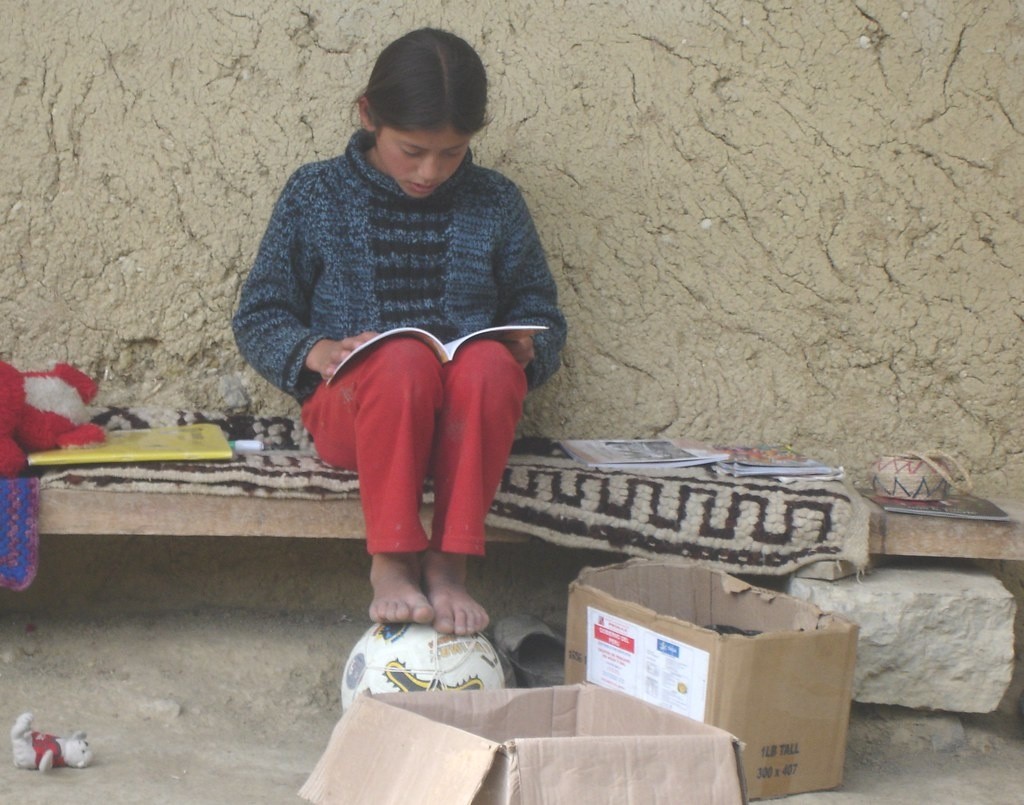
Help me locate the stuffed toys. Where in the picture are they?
[10,711,95,774]
[0,362,108,476]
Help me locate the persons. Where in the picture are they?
[233,26,568,641]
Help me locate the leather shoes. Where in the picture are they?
[492,612,565,686]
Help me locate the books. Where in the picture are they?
[557,434,845,485]
[24,421,233,467]
[323,316,550,393]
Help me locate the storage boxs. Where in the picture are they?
[561,558,860,798]
[293,682,753,804]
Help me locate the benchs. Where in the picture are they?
[1,441,1021,588]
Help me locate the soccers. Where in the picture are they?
[341,622,505,715]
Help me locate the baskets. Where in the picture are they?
[872,451,949,502]
